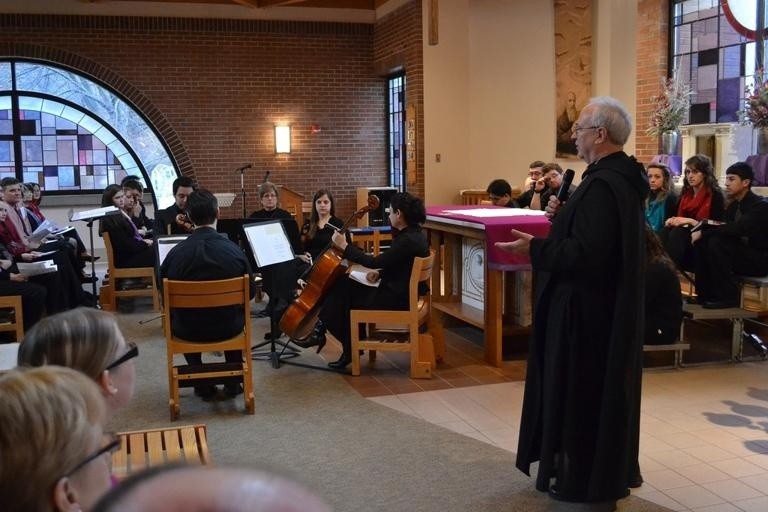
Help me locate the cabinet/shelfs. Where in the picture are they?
[356,187,398,229]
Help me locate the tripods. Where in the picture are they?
[251,273,301,353]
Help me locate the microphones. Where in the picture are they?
[236,165,253,170]
[556,169,575,202]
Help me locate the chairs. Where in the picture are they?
[0,295,27,344]
[348,247,447,380]
[163,273,256,424]
[98,230,160,313]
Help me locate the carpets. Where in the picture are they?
[0,262,683,511]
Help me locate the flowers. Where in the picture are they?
[642,50,698,140]
[734,52,768,136]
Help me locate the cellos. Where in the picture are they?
[278,194,379,340]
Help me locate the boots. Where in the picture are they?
[329,351,363,367]
[291,321,327,353]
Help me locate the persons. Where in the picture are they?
[528,162,578,209]
[664,153,725,275]
[515,160,547,208]
[493,96,652,504]
[486,178,520,208]
[644,154,678,255]
[686,161,768,309]
[557,92,584,155]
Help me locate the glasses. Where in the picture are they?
[107,342,138,370]
[576,125,597,133]
[67,432,122,475]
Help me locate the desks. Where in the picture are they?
[345,225,393,256]
[419,203,552,370]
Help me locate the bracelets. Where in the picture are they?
[534,188,541,193]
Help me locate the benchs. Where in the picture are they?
[110,424,214,483]
[641,187,767,371]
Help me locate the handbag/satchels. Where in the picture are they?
[695,230,737,306]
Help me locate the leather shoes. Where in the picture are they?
[83,255,100,261]
[81,274,98,283]
[223,385,242,395]
[195,384,216,394]
[551,486,591,502]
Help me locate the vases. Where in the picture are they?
[662,127,679,156]
[756,123,768,153]
